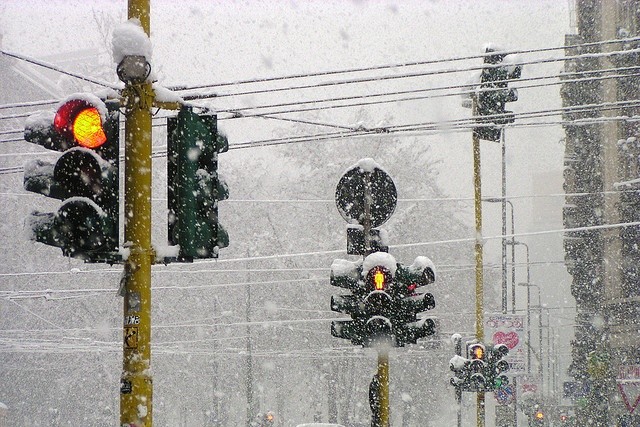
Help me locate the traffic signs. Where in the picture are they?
[484,313,524,331]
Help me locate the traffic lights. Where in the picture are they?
[395,265,435,347]
[24,112,65,250]
[330,264,363,345]
[53,95,119,263]
[474,48,520,141]
[363,265,396,346]
[467,345,488,390]
[489,344,509,390]
[450,355,467,389]
